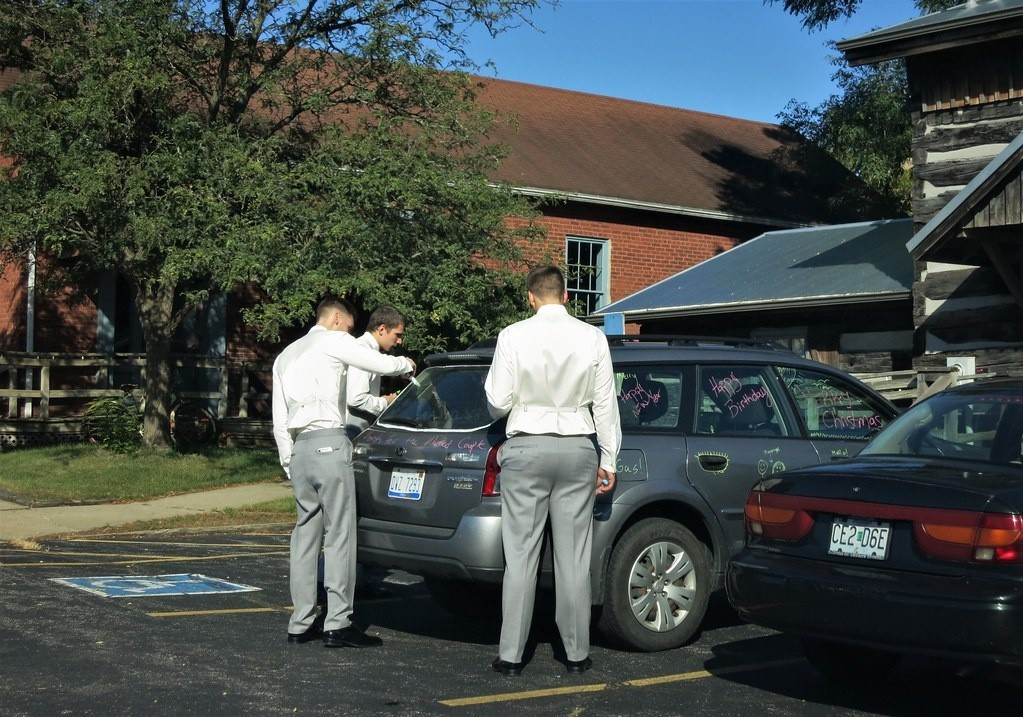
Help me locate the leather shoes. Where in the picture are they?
[323,626,383,649]
[288,620,324,643]
[568,656,593,674]
[492,657,522,677]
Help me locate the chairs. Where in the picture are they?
[731,383,781,434]
[620,376,668,425]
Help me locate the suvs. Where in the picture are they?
[353,334,1002,654]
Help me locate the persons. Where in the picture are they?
[272,296,417,649]
[345,305,407,442]
[484,265,622,677]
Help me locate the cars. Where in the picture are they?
[723,364,1023,675]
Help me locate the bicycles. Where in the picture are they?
[84,383,218,451]
